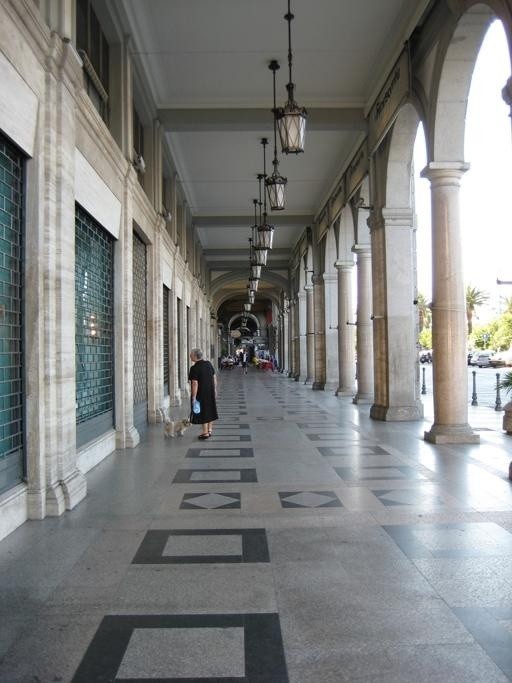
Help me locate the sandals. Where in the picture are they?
[199,431,212,439]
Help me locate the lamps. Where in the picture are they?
[243,0,308,312]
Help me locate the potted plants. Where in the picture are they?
[494,372,512,434]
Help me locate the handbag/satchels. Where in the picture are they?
[193,401,200,414]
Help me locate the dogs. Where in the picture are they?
[163,416,192,438]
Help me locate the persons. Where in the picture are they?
[240,349,248,375]
[187,347,218,441]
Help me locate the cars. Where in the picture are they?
[418,347,512,367]
